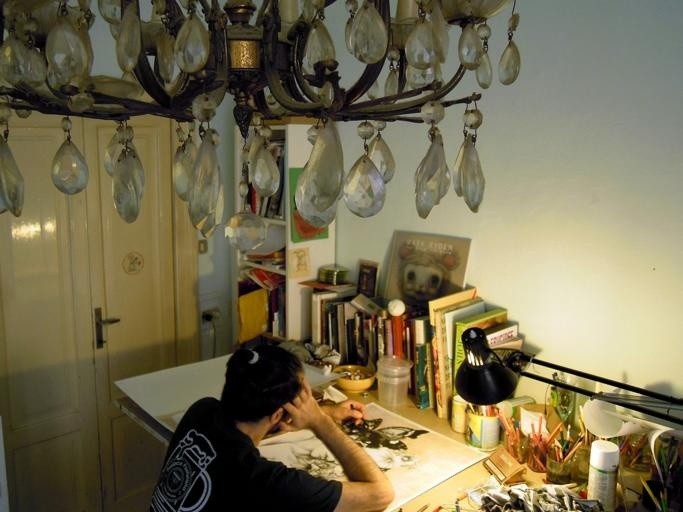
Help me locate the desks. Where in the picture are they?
[111,354,546,512]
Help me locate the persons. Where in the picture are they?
[148,337,395,511]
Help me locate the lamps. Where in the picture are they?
[454,327,683,432]
[0,1,521,255]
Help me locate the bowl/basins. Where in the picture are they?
[333,366,375,393]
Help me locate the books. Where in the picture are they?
[240,139,287,337]
[306,286,524,419]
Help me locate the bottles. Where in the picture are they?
[586,439,620,510]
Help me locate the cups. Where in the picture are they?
[450,396,575,485]
[375,375,409,408]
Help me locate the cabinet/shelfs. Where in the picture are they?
[232,117,335,343]
[0,75,203,511]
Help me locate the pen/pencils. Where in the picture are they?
[639,436,683,512]
[618,435,646,466]
[464,403,498,416]
[528,411,586,475]
[495,410,522,463]
[350,404,370,428]
